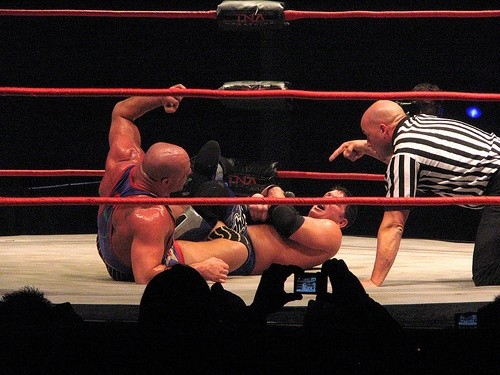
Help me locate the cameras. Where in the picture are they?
[294,273,327,294]
[455,312,477,326]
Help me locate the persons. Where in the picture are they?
[329,97,500,289]
[0,83,500,375]
